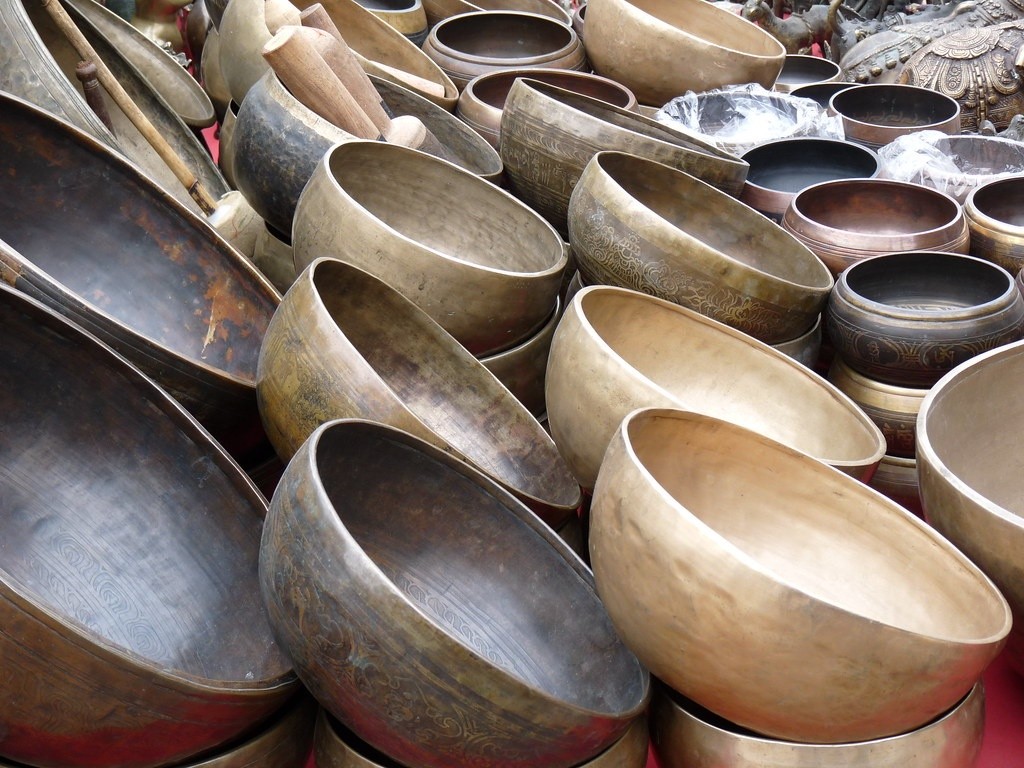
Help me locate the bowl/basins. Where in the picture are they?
[0,1,1023,767]
